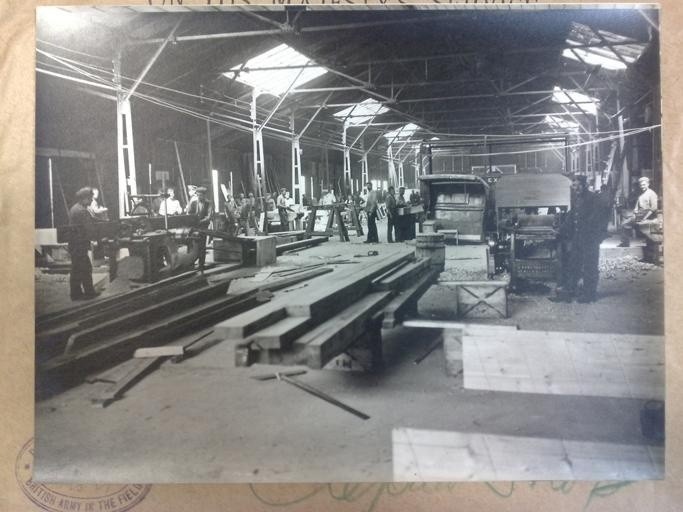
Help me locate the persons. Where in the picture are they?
[87,188,108,217]
[68,187,101,300]
[224,188,294,231]
[547,174,608,304]
[617,177,658,247]
[152,185,213,267]
[319,183,420,243]
